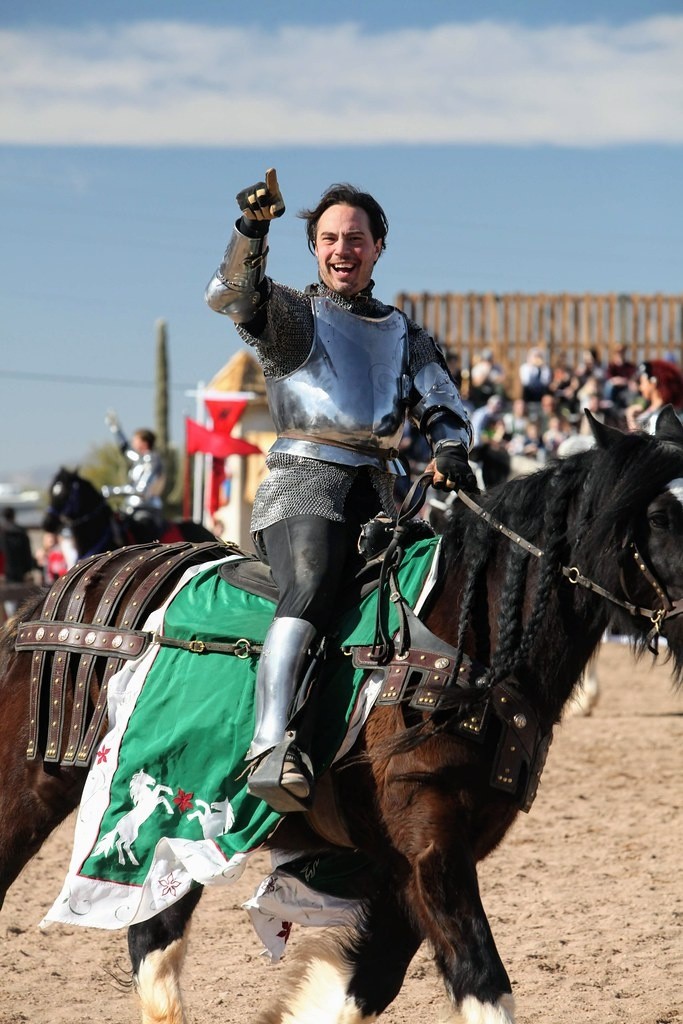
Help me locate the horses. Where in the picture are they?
[1,406,682,1024]
[40,465,219,564]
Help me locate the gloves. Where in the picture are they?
[235,166,286,224]
[425,448,479,494]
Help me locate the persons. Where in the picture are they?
[444,338,683,496]
[209,164,476,804]
[1,506,68,619]
[103,403,172,546]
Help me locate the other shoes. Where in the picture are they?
[247,745,310,799]
